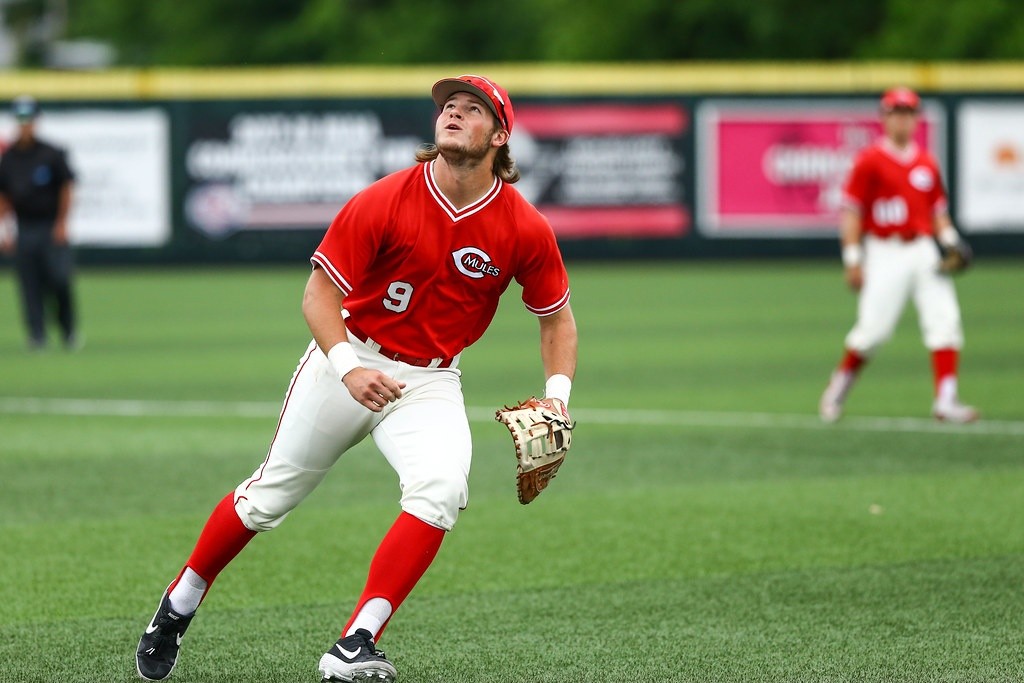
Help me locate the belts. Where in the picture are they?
[872,231,918,241]
[344,316,453,368]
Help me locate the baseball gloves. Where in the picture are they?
[936,242,974,276]
[496,397,574,507]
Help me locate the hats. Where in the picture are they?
[432,75,514,135]
[14,101,40,122]
[882,88,919,108]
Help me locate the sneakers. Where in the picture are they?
[319,628,397,683]
[136,578,197,682]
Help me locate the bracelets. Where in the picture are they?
[840,243,863,267]
[937,225,963,250]
[327,342,364,382]
[545,373,572,409]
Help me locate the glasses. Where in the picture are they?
[457,74,509,131]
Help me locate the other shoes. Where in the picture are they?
[820,368,852,422]
[932,402,978,422]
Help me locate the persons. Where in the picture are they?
[132,75,578,683]
[0,93,80,351]
[816,84,979,425]
